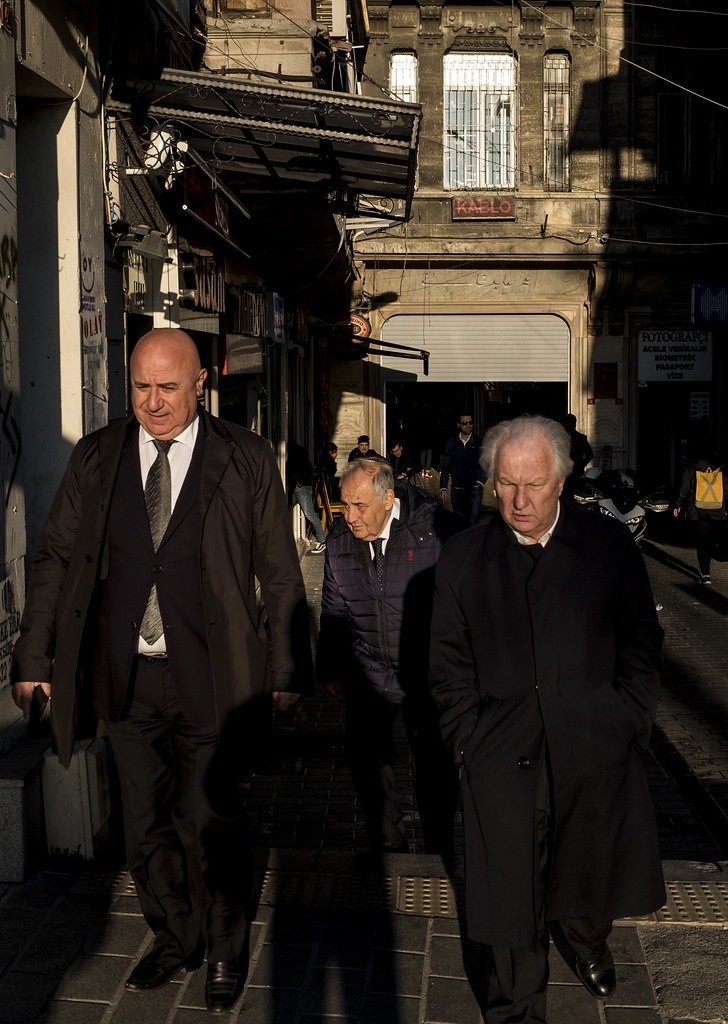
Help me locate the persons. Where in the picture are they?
[348,436,390,463]
[562,414,595,480]
[674,443,728,584]
[320,441,338,502]
[388,440,422,479]
[9,326,315,1016]
[317,457,473,878]
[427,415,667,1023]
[287,440,326,553]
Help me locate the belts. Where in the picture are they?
[138,654,167,662]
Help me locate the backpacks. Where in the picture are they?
[694,465,723,510]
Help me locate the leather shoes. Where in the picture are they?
[125,943,208,993]
[572,940,616,999]
[205,953,249,1016]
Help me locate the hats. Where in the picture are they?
[357,435,369,445]
[328,442,337,452]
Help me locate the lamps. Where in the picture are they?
[118,229,174,264]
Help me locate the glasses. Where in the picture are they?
[459,420,473,426]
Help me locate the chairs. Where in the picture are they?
[315,479,346,533]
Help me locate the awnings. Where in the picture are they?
[102,62,423,222]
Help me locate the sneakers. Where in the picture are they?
[697,573,712,584]
[310,540,327,554]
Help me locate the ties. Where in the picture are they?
[370,538,386,592]
[138,438,177,647]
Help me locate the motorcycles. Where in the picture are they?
[573,465,673,547]
